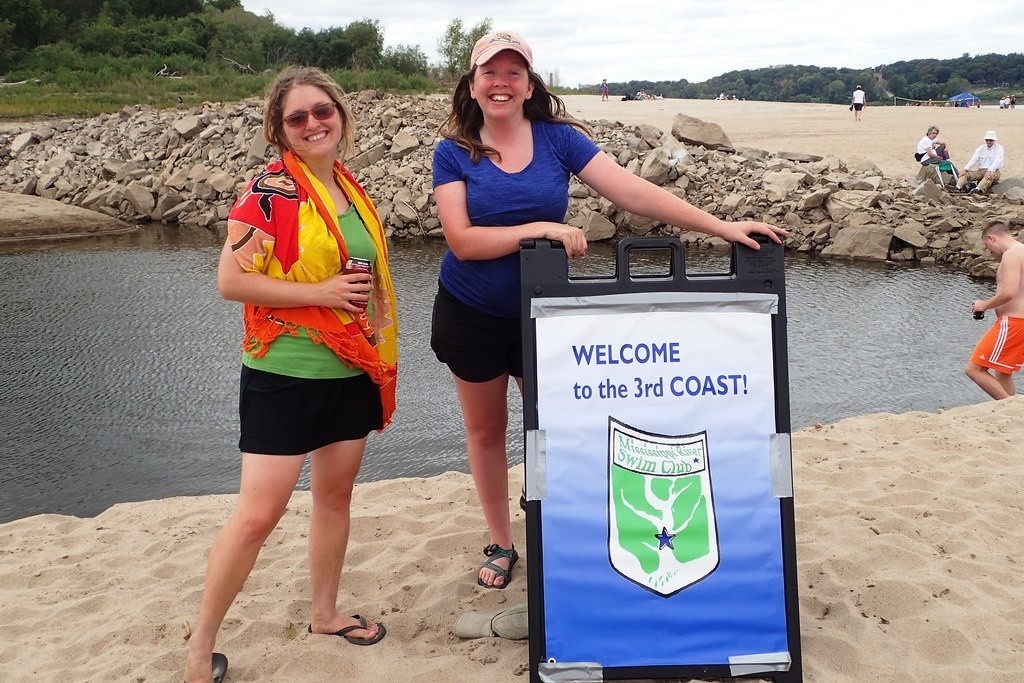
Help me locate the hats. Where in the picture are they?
[985,131,998,140]
[471,31,533,72]
[454,605,528,640]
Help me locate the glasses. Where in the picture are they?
[986,140,993,141]
[282,102,336,129]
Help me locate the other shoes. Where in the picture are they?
[945,184,960,193]
[967,188,980,196]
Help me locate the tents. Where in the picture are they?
[949,92,979,101]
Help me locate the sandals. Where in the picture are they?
[478,543,519,589]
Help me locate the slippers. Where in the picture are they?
[212,653,228,683]
[308,614,386,646]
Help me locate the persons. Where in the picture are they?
[427,30,788,590]
[999,95,1016,110]
[945,131,1004,195]
[918,126,959,176]
[635,88,663,100]
[852,84,867,121]
[182,66,398,683]
[955,99,981,108]
[720,91,739,101]
[964,220,1024,400]
[600,79,608,102]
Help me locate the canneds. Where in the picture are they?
[342,256,373,312]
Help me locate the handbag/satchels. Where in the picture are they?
[915,153,926,162]
